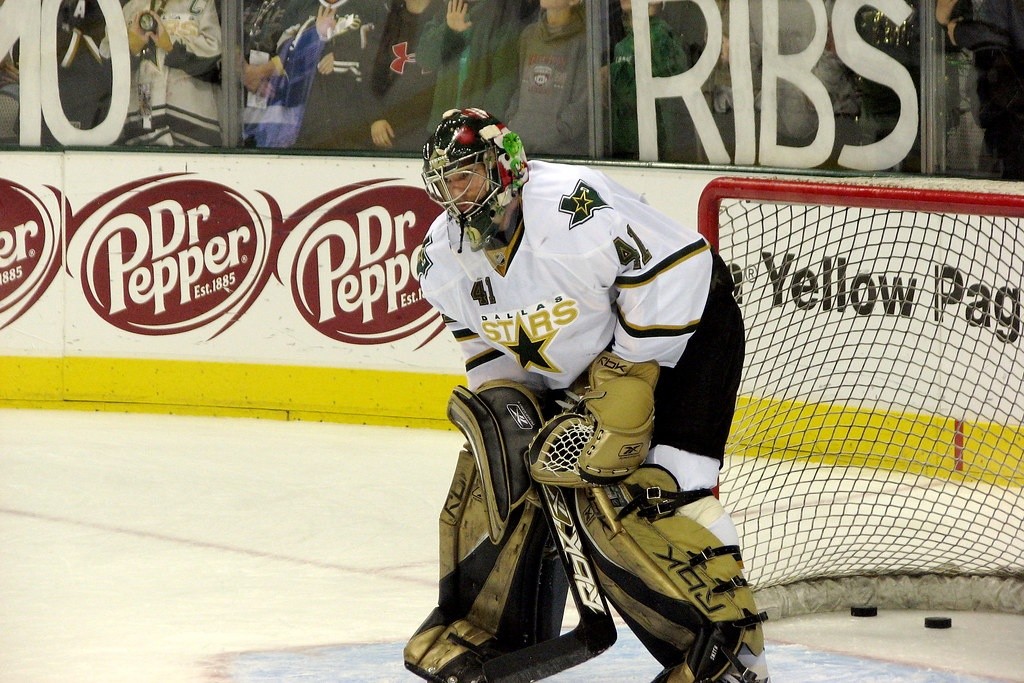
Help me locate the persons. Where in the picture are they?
[0,0,1024,183]
[407,108,771,683]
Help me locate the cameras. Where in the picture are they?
[140,14,157,32]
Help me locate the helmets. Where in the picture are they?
[419,105,528,251]
